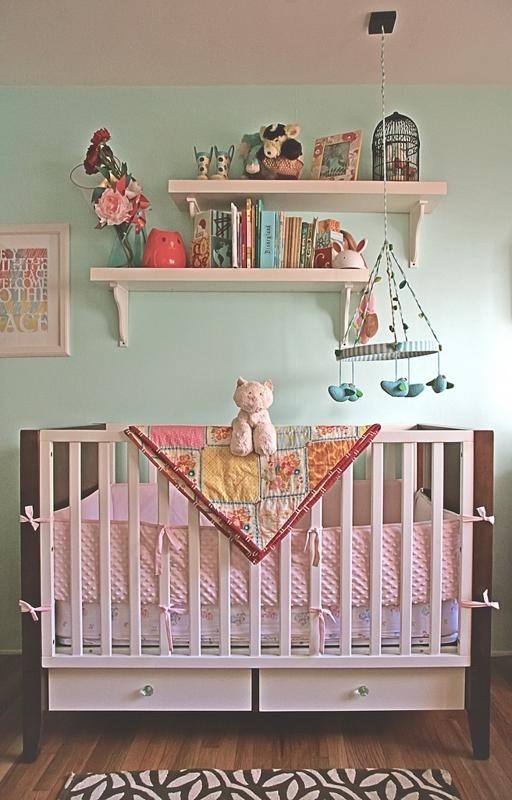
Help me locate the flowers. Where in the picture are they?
[84,127,152,237]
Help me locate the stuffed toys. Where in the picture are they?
[237,122,303,179]
[232,376,275,456]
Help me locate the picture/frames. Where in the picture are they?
[307,128,364,181]
[0,221,71,359]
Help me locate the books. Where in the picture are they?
[191,197,319,268]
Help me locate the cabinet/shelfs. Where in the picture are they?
[86,177,449,349]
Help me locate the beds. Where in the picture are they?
[17,421,496,760]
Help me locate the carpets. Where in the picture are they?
[55,766,464,800]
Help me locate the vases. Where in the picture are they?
[107,223,150,268]
